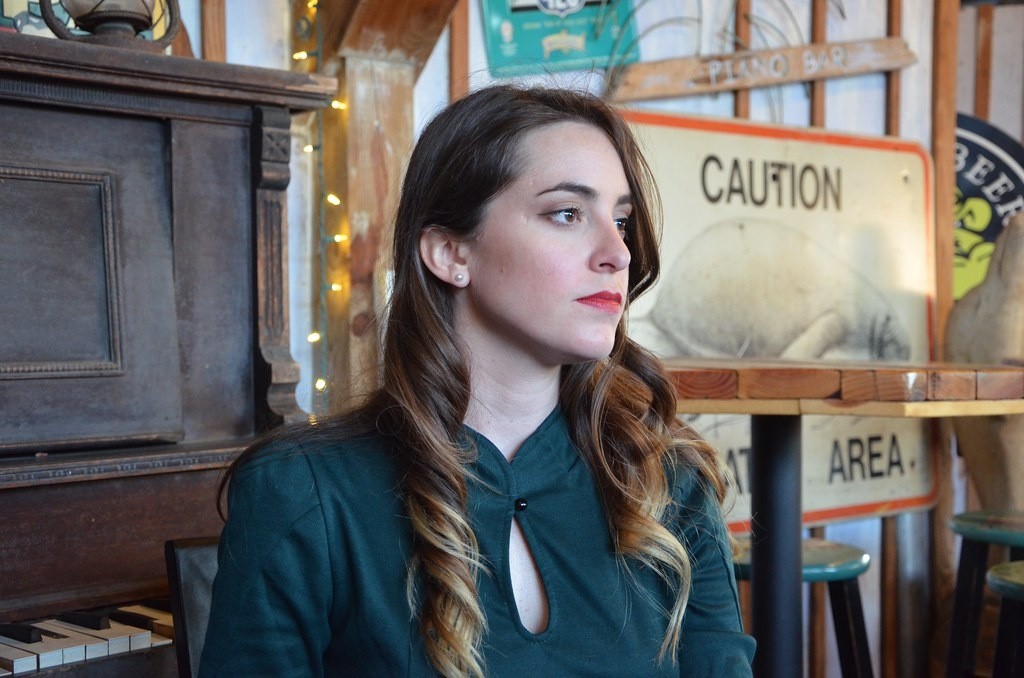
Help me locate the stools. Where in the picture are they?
[733,537,874,678]
[946,509,1024,678]
[986,560,1024,678]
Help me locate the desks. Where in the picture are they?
[627,361,1024,678]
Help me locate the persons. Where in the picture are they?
[197,83,757,678]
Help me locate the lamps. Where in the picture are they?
[292,0,348,427]
[40,0,178,55]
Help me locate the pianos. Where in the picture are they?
[0,28,340,678]
